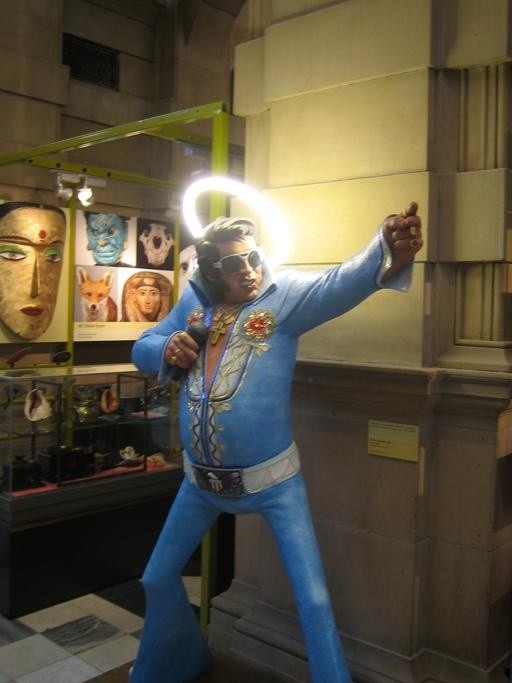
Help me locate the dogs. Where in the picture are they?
[75,265,119,323]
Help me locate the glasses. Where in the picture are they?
[211,249,262,275]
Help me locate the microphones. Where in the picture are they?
[172,320,208,381]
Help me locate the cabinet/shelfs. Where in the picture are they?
[1,362,202,618]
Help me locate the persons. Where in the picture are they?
[85,213,131,266]
[0,203,65,339]
